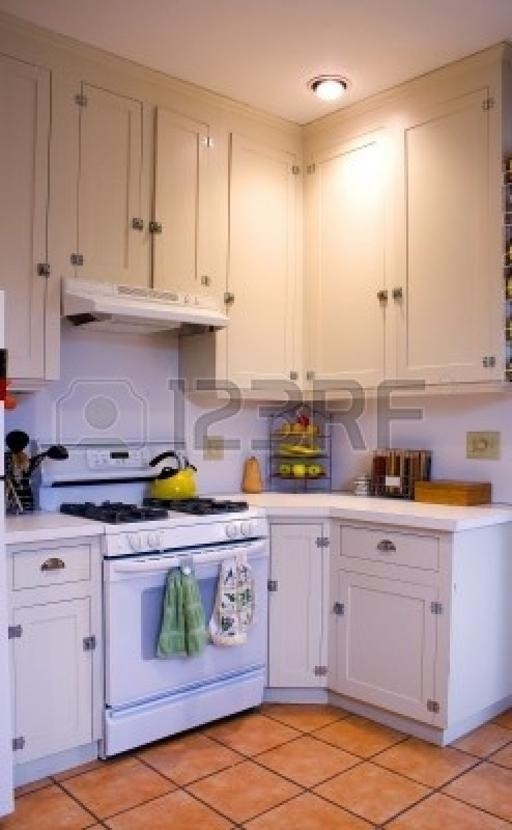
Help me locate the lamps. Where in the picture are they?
[308,76,349,103]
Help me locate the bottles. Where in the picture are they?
[354,473,373,496]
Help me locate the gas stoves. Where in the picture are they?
[57,495,268,536]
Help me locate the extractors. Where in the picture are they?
[58,275,232,337]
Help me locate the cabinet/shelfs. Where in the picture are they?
[214,132,304,389]
[68,77,209,298]
[1,48,60,393]
[1,513,105,764]
[330,513,512,730]
[268,517,328,690]
[305,75,496,393]
[265,401,335,493]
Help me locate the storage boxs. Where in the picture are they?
[414,480,492,506]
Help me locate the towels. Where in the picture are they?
[155,566,209,657]
[207,550,256,649]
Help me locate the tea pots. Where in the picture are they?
[241,457,265,494]
[147,451,199,503]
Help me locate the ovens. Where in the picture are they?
[104,518,271,759]
